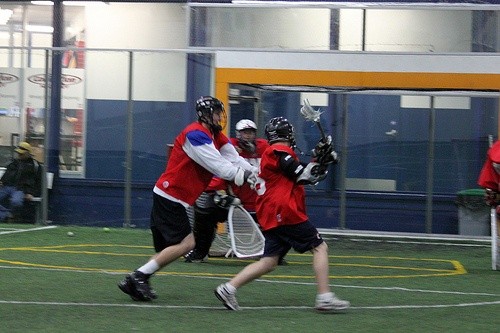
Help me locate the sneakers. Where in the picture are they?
[214,283,242,311]
[129,269,156,298]
[118,276,152,301]
[315,294,350,311]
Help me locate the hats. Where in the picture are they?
[14,141,32,154]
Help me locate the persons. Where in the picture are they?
[214,116,350,311]
[166,119,286,264]
[117,95,259,302]
[477,140,500,270]
[0,142,42,222]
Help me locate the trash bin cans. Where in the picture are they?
[456,188,490,236]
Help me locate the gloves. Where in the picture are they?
[234,167,258,186]
[311,136,338,170]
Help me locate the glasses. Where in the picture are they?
[17,146,30,152]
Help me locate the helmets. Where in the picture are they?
[236,119,257,148]
[264,117,295,143]
[196,97,226,132]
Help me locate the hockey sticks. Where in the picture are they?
[226,183,265,259]
[301,98,326,140]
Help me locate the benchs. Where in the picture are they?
[0,168,53,224]
